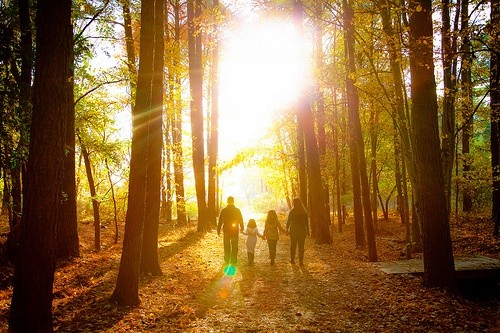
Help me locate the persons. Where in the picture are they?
[240,218,263,266]
[262,210,288,265]
[216,196,244,265]
[286,198,311,265]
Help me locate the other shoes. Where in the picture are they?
[249,260,255,264]
[299,260,303,263]
[231,260,237,264]
[271,259,274,265]
[289,260,295,264]
[225,259,229,263]
